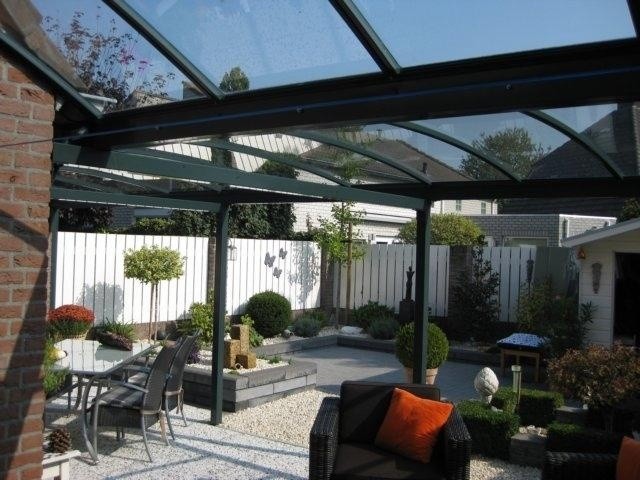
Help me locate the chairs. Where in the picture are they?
[306,380,474,479]
[544,429,640,479]
[119,331,202,439]
[87,337,181,460]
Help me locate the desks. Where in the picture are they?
[497,332,552,381]
[39,338,157,464]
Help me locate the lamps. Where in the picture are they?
[574,247,586,266]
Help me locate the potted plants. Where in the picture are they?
[394,320,450,386]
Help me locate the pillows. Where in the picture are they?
[616,431,639,479]
[375,386,453,466]
